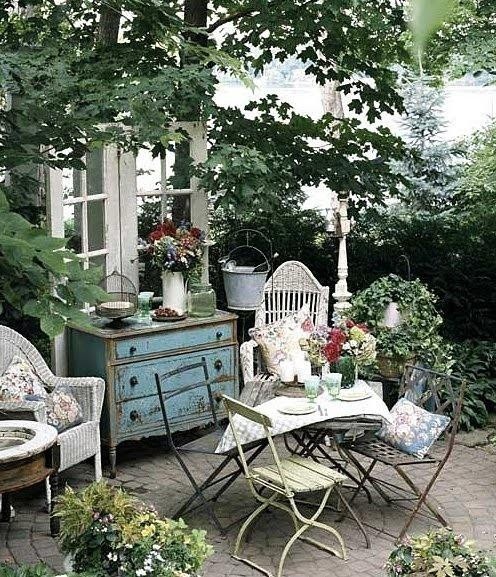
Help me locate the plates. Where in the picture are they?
[150,314,188,322]
[277,401,318,415]
[334,388,374,402]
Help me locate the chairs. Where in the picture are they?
[337,368,466,554]
[219,393,348,577]
[0,324,105,514]
[154,356,269,535]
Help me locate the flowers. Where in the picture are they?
[322,320,377,360]
[141,220,211,271]
[50,480,217,577]
[382,529,494,577]
[296,328,331,361]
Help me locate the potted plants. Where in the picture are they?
[351,279,442,379]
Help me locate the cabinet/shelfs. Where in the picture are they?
[64,308,241,478]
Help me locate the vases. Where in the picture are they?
[327,358,360,390]
[141,272,214,316]
[310,361,328,380]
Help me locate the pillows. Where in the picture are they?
[248,301,327,379]
[26,379,86,432]
[0,352,47,406]
[375,397,449,460]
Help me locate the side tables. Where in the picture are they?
[0,419,63,538]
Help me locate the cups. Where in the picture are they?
[326,373,342,401]
[303,378,319,403]
